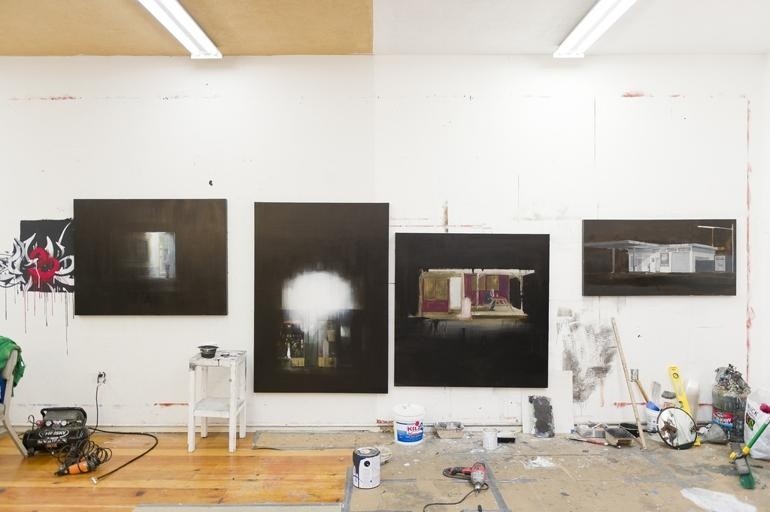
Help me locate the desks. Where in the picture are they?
[188,349,248,453]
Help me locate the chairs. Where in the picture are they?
[0,349,29,458]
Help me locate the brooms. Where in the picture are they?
[728,416,770,491]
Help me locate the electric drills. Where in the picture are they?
[54,452,95,476]
[443,462,485,497]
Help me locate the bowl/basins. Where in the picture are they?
[197,345,218,358]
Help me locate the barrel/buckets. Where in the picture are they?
[483,427,498,451]
[352,447,381,489]
[645,406,662,433]
[393,403,425,445]
